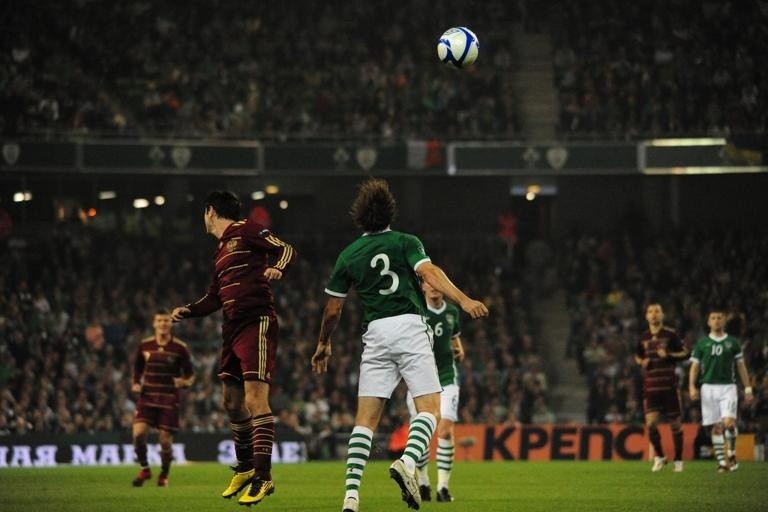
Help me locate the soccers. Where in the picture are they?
[437,26,480,66]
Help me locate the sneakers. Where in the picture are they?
[717,455,739,473]
[132,468,169,487]
[651,455,667,472]
[437,486,453,502]
[238,476,275,507]
[222,464,255,498]
[342,498,359,512]
[672,460,683,472]
[419,484,432,501]
[389,459,421,510]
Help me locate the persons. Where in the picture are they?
[630,298,694,474]
[128,307,199,490]
[311,176,491,511]
[170,188,299,508]
[404,266,467,505]
[0,0,768,436]
[686,304,756,475]
